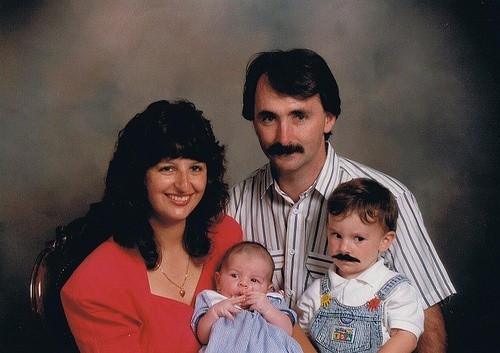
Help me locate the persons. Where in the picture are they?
[59,96,240,352]
[188,239,302,353]
[223,46,456,353]
[292,176,424,353]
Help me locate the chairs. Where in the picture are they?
[19,200,111,353]
